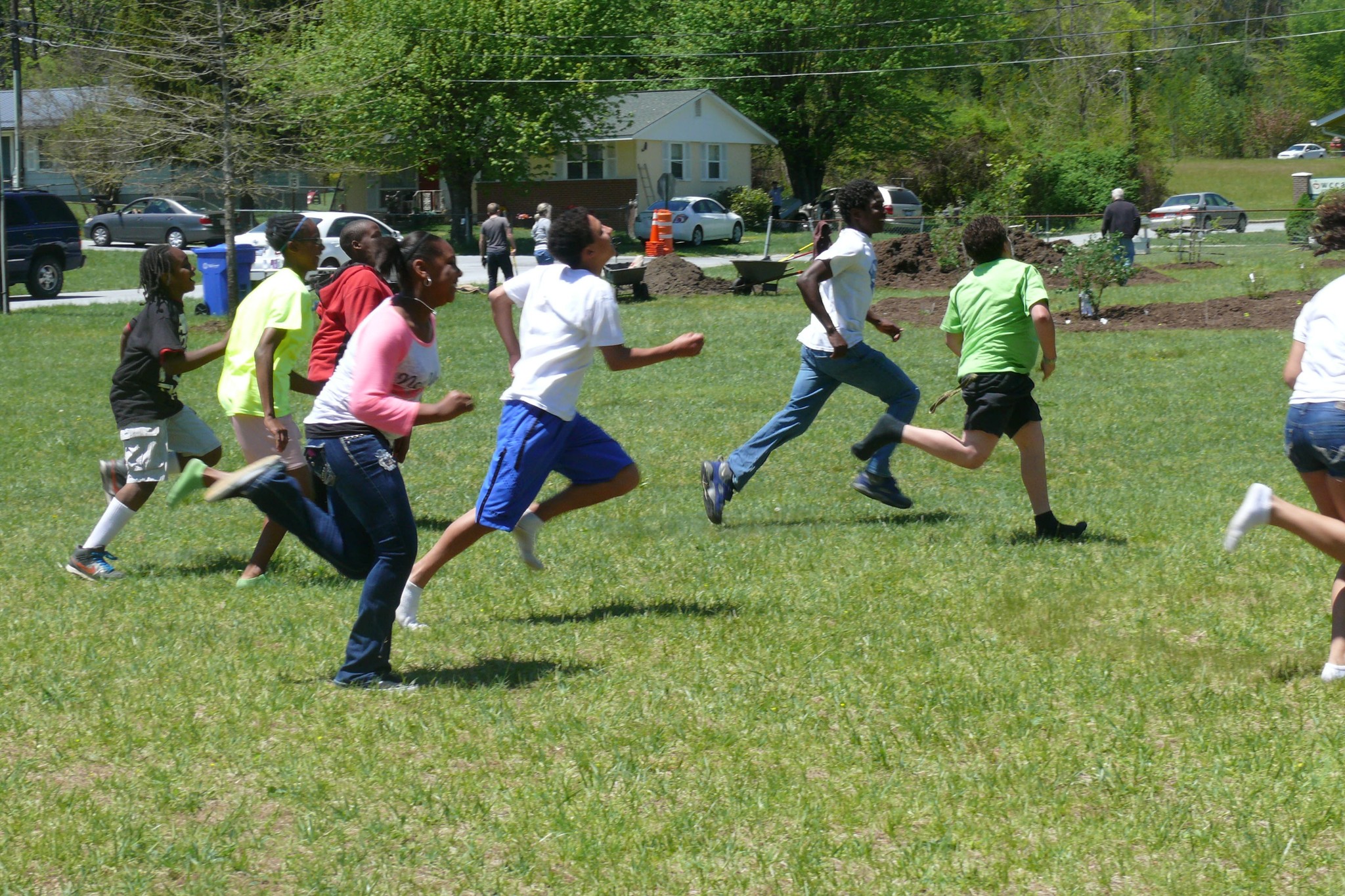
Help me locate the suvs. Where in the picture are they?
[0,185,86,300]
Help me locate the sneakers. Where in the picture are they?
[66,545,126,584]
[701,456,733,524]
[204,453,287,502]
[334,674,420,694]
[99,458,128,505]
[853,469,912,509]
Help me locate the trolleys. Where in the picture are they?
[728,259,805,297]
[603,262,649,300]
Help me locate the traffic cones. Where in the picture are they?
[645,209,664,257]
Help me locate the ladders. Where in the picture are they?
[637,163,657,207]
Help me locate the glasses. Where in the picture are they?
[292,238,323,245]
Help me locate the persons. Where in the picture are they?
[1332,136,1341,155]
[813,211,833,261]
[60,244,234,583]
[165,218,402,514]
[1221,275,1345,684]
[204,230,477,696]
[852,214,1087,542]
[768,180,787,219]
[393,209,705,634]
[132,201,149,215]
[531,203,555,265]
[701,176,921,527]
[1101,188,1141,286]
[216,210,333,591]
[479,203,517,300]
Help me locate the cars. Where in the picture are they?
[797,186,849,232]
[876,185,923,230]
[1148,192,1248,238]
[84,195,242,250]
[1276,143,1327,160]
[633,196,745,248]
[234,211,405,290]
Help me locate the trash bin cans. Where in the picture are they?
[190,243,257,315]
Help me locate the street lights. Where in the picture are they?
[1107,66,1142,141]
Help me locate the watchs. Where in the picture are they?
[1043,353,1057,365]
